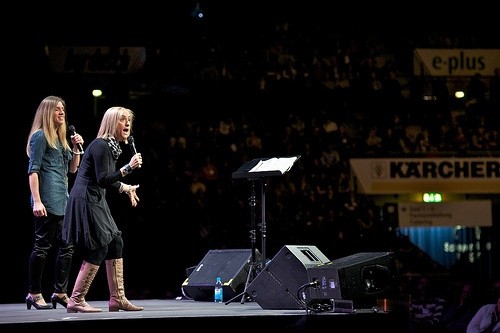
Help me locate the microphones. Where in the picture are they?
[67,125,84,154]
[127,136,142,168]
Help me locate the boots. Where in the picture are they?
[66,260,102,313]
[105,258,144,311]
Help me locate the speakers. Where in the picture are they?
[181,248,401,310]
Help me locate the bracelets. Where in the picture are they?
[123,164,133,175]
[73,148,82,155]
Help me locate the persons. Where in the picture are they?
[26,95,84,310]
[62,106,144,313]
[442,284,480,333]
[464,297,500,333]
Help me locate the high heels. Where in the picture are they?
[26,293,52,309]
[51,294,68,309]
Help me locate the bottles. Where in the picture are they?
[214,277,223,303]
